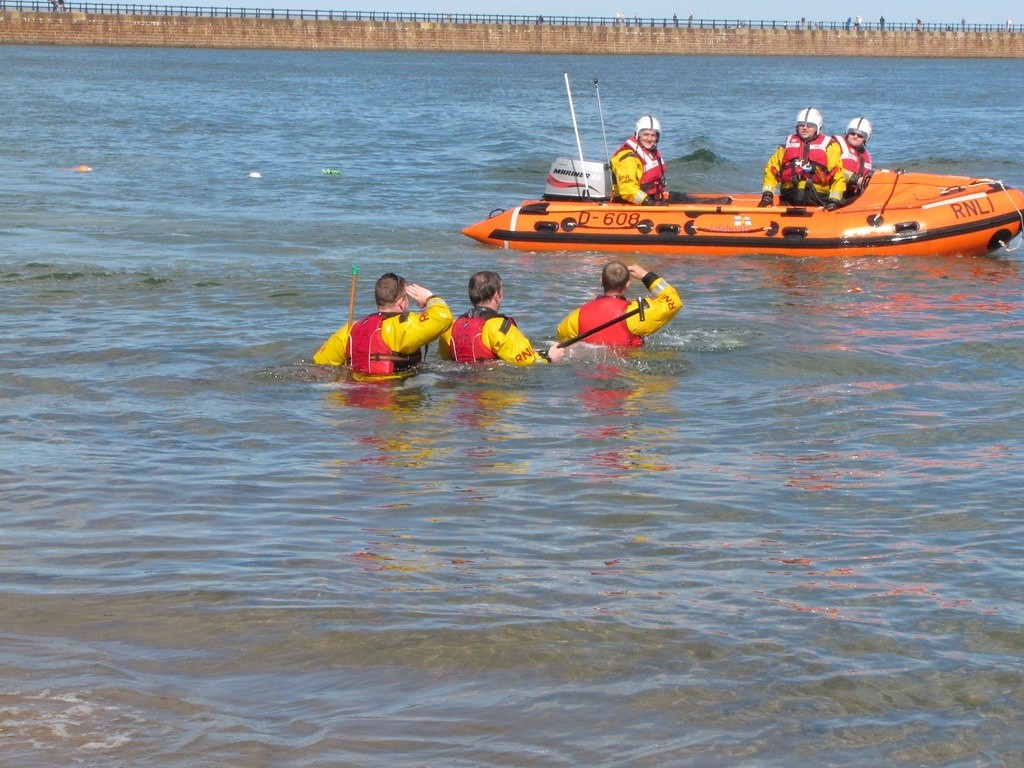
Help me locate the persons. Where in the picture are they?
[313,273,453,380]
[915,17,923,32]
[961,17,966,31]
[50,0,67,12]
[558,261,683,348]
[536,14,543,25]
[1005,18,1011,32]
[672,13,677,27]
[757,107,872,211]
[783,16,819,30]
[439,272,565,366]
[845,15,885,31]
[688,10,693,27]
[610,115,668,206]
[616,11,626,26]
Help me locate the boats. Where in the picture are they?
[462,71,1024,256]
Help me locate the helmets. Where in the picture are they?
[634,115,660,142]
[845,117,872,143]
[795,107,821,136]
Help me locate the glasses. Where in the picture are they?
[850,132,863,138]
[393,273,404,301]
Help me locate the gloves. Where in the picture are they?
[758,191,774,208]
[647,199,667,207]
[824,202,836,210]
